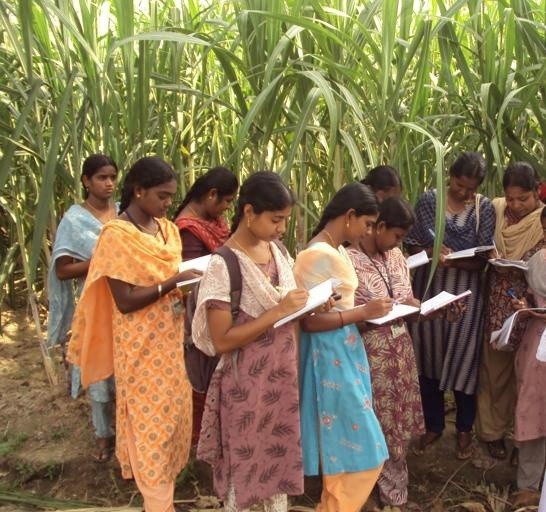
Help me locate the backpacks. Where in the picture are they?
[182,239,288,394]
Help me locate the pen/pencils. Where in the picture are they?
[372,296,399,306]
[275,287,286,296]
[429,228,435,236]
[493,239,498,255]
[508,290,519,301]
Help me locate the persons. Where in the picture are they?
[361,161,404,207]
[168,165,240,459]
[188,170,342,512]
[474,163,546,467]
[344,196,467,511]
[490,209,546,512]
[402,152,496,463]
[66,155,204,512]
[285,182,398,511]
[43,154,124,462]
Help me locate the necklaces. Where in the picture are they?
[232,235,274,283]
[125,208,161,237]
[322,230,347,260]
[84,200,118,223]
[445,198,468,218]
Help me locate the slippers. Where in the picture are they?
[488,439,508,458]
[411,431,445,457]
[90,445,110,463]
[510,447,519,467]
[455,436,475,459]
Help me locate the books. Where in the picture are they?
[420,288,473,316]
[485,253,530,271]
[353,302,420,326]
[407,248,429,269]
[176,251,212,287]
[443,244,495,260]
[490,306,546,350]
[273,276,340,328]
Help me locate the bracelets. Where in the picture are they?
[336,310,345,330]
[157,282,164,299]
